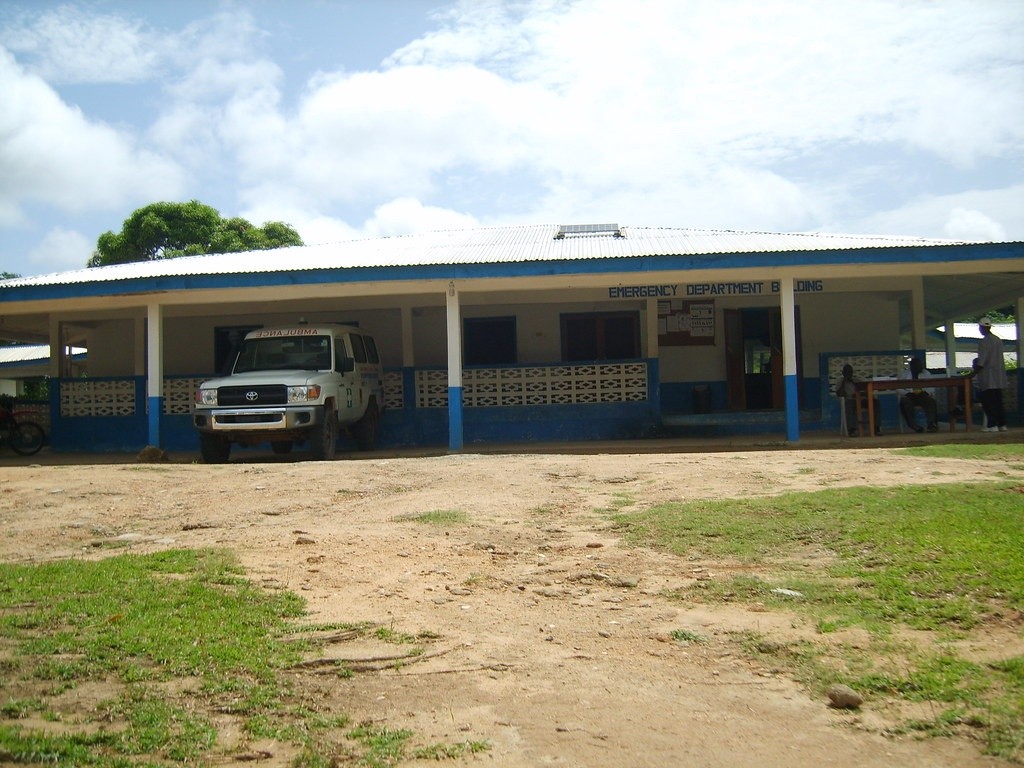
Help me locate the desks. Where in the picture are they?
[854,374,973,437]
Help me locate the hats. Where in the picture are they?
[979,317,991,327]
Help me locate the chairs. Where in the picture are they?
[834,375,878,436]
[895,369,936,433]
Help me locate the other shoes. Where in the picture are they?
[927,425,937,432]
[916,425,924,433]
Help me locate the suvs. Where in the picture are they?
[194,325,382,462]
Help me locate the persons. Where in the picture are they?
[971,316,1008,432]
[953,357,982,412]
[895,358,939,432]
[835,364,882,436]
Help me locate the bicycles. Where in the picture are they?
[0,410,45,455]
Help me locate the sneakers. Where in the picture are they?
[981,425,1008,433]
